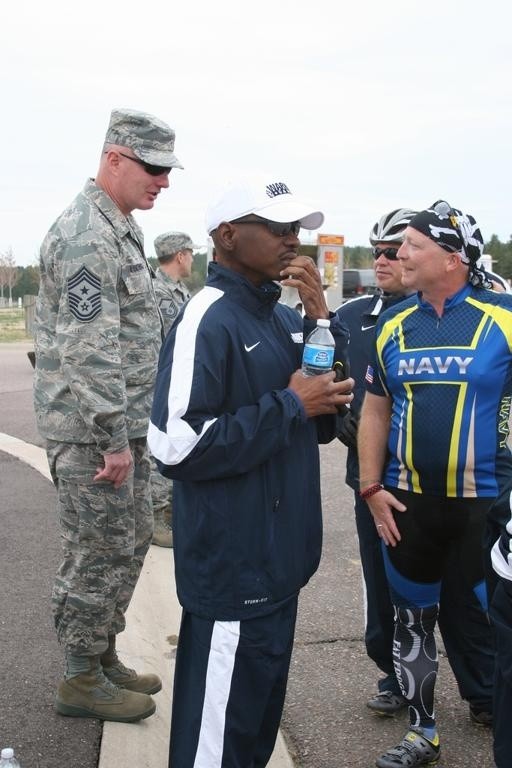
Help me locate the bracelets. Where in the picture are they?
[355,480,385,501]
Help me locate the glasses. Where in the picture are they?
[118,153,171,177]
[371,246,399,260]
[236,219,303,237]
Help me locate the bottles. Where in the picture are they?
[301,318,336,377]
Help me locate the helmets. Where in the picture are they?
[368,208,419,246]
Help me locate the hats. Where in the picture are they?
[105,108,183,169]
[155,230,204,257]
[207,181,324,234]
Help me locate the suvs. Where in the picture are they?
[341,268,376,302]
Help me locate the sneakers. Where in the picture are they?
[470,707,493,728]
[376,729,445,767]
[366,689,407,716]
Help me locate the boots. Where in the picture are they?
[55,654,156,722]
[101,634,162,693]
[152,512,175,548]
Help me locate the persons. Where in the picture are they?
[357,199,511,767]
[149,229,202,550]
[144,171,354,767]
[330,207,512,728]
[30,110,185,722]
[477,481,512,768]
